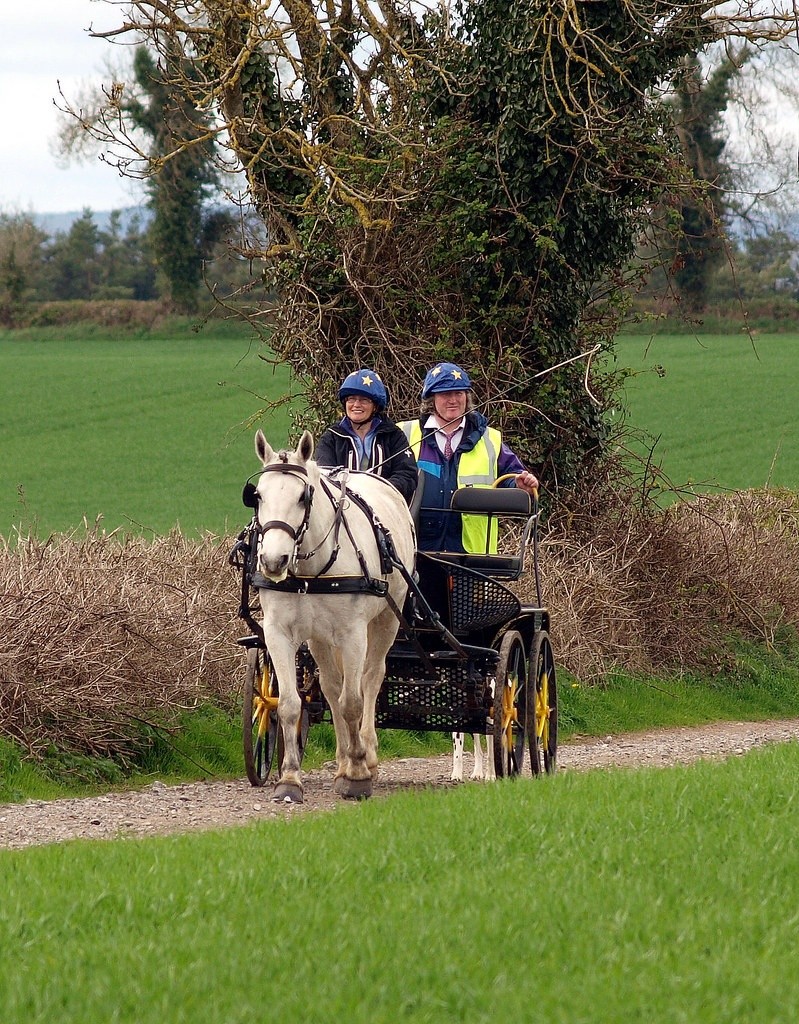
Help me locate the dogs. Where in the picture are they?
[446,671,511,784]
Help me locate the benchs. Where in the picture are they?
[409,468,538,581]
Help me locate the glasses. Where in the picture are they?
[348,398,370,404]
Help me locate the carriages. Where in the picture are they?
[227,429,559,804]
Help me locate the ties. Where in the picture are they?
[438,427,462,459]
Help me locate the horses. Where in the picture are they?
[254,428,418,805]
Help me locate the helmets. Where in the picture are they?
[422,362,471,399]
[339,369,386,416]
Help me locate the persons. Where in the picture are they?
[316,370,418,495]
[395,363,539,573]
